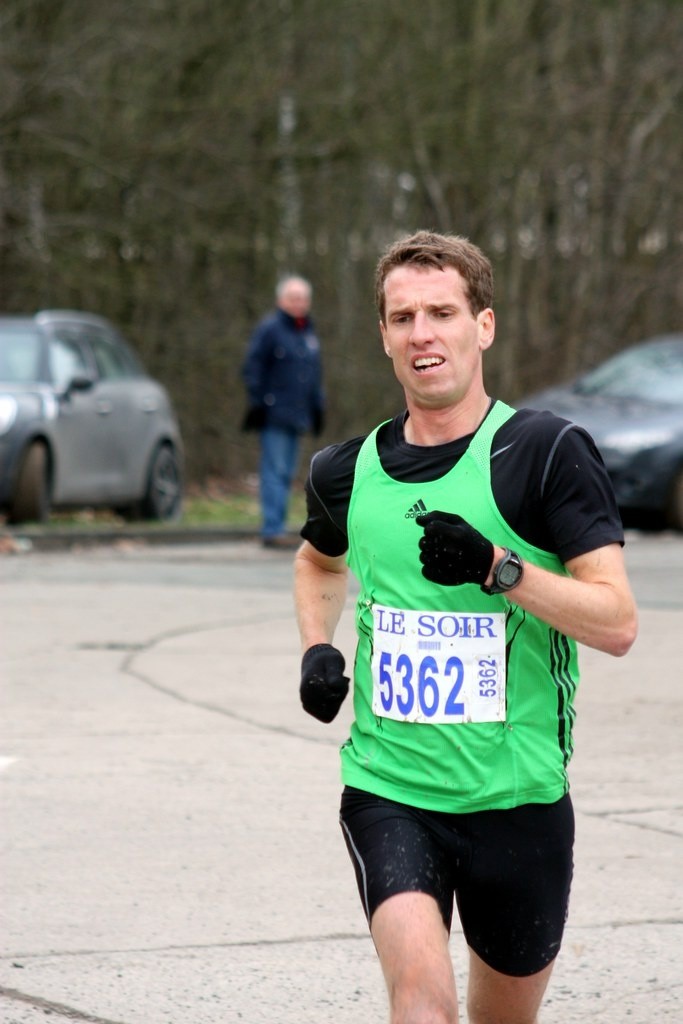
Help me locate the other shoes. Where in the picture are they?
[262,532,305,551]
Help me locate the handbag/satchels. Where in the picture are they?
[238,400,277,437]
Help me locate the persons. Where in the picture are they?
[293,227,642,1024]
[238,273,330,549]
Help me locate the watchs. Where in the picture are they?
[482,548,524,596]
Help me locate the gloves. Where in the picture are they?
[415,510,495,586]
[300,643,350,724]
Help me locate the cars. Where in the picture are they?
[509,335,683,534]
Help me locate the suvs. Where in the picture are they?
[0,310,186,526]
